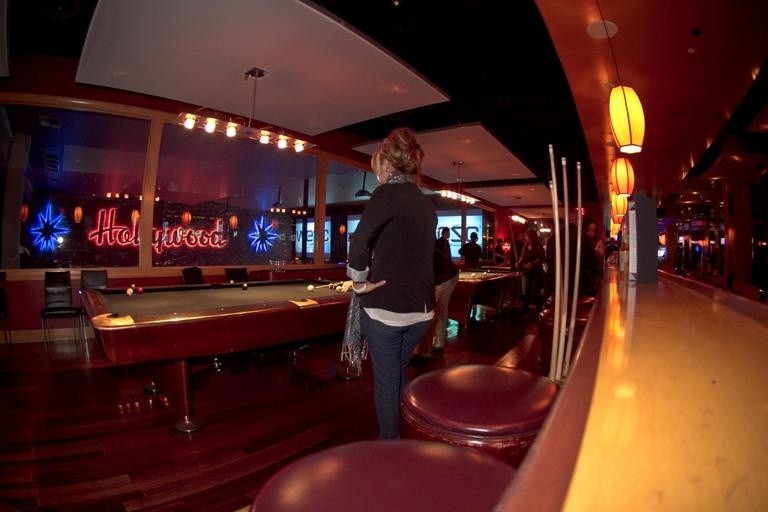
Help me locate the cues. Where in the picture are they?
[510,219,518,261]
[547,144,582,381]
[316,282,343,288]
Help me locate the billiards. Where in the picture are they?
[126,288,133,296]
[329,283,342,293]
[307,285,314,291]
[136,287,144,294]
[242,283,248,290]
[484,272,486,274]
[472,272,475,276]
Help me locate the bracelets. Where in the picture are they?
[351,282,366,293]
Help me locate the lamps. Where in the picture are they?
[539,227,551,232]
[441,177,481,204]
[606,80,648,242]
[17,206,240,232]
[511,213,529,224]
[355,171,371,197]
[102,190,161,202]
[338,224,346,236]
[269,185,309,216]
[174,67,318,154]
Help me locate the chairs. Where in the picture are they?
[225,267,248,282]
[40,269,108,365]
[183,267,205,284]
[0,272,12,344]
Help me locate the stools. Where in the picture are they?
[398,363,556,447]
[249,440,515,511]
[537,292,596,376]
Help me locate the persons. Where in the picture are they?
[516,225,555,322]
[438,227,452,257]
[458,232,483,270]
[495,238,506,265]
[341,247,459,352]
[488,240,493,258]
[346,126,435,440]
